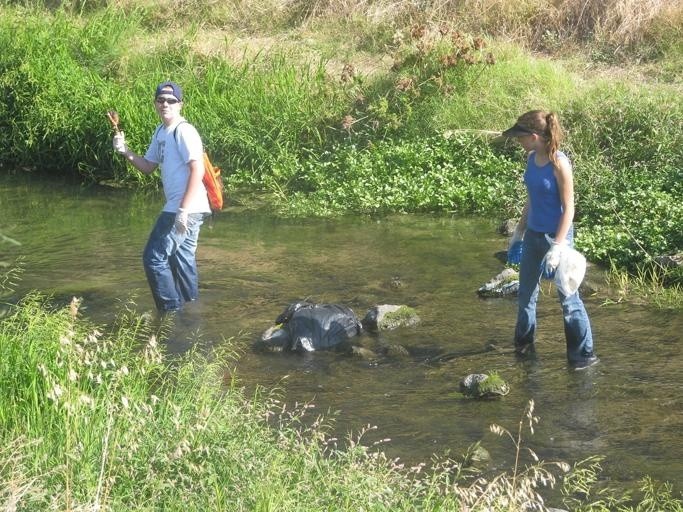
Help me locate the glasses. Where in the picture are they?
[156,97,178,104]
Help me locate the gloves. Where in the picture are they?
[175,209,188,231]
[113,131,126,153]
[507,232,524,264]
[540,242,560,279]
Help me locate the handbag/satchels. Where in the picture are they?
[202,152,224,209]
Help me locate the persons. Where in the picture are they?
[112,82,213,316]
[502,110,600,372]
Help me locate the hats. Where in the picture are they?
[502,123,547,137]
[156,83,182,101]
